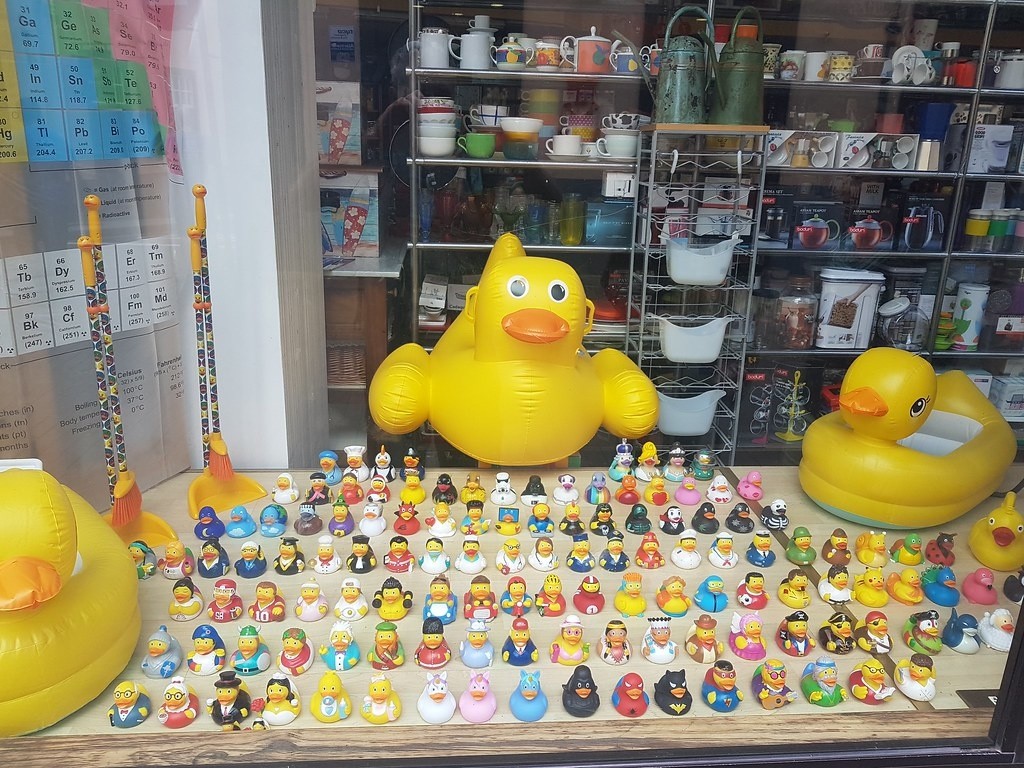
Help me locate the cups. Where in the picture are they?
[961,208,1024,255]
[767,102,915,172]
[711,19,1024,89]
[417,13,663,76]
[458,88,641,163]
[418,181,584,246]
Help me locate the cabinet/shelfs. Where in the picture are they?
[365,0,1024,468]
[323,236,411,468]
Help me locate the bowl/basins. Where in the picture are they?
[416,96,457,158]
[641,114,753,167]
[767,143,788,165]
[846,145,870,168]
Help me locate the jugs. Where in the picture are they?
[785,138,811,167]
[765,208,787,240]
[867,136,895,168]
[904,203,944,250]
[850,214,894,249]
[918,100,957,139]
[797,214,840,248]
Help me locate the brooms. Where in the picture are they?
[192,183,236,482]
[83,193,143,529]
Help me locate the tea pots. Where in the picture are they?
[697,5,764,126]
[611,4,715,124]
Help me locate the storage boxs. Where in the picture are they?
[934,369,993,398]
[748,184,799,250]
[912,292,956,344]
[887,188,947,253]
[421,274,450,296]
[822,384,842,412]
[319,168,390,258]
[637,205,690,246]
[447,284,475,310]
[834,132,920,170]
[600,170,635,204]
[317,81,368,166]
[944,123,1014,174]
[720,359,775,448]
[771,359,825,446]
[980,359,1024,376]
[848,180,885,205]
[639,182,689,208]
[988,376,1024,418]
[789,201,843,251]
[698,176,751,209]
[843,201,900,252]
[692,208,753,248]
[419,294,446,309]
[582,200,634,248]
[752,130,838,169]
[1005,118,1024,175]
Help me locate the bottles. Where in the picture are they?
[725,262,1024,353]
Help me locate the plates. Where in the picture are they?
[545,153,591,163]
[597,156,638,162]
[848,76,893,85]
[891,45,926,69]
